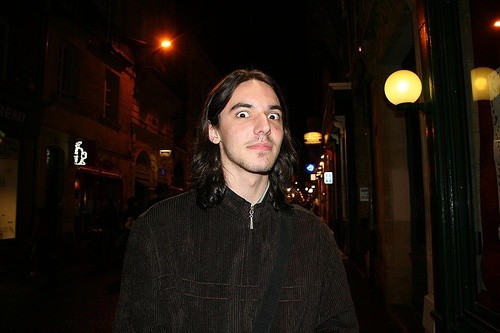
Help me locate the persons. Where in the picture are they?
[113,68,360,333]
[96,182,170,279]
